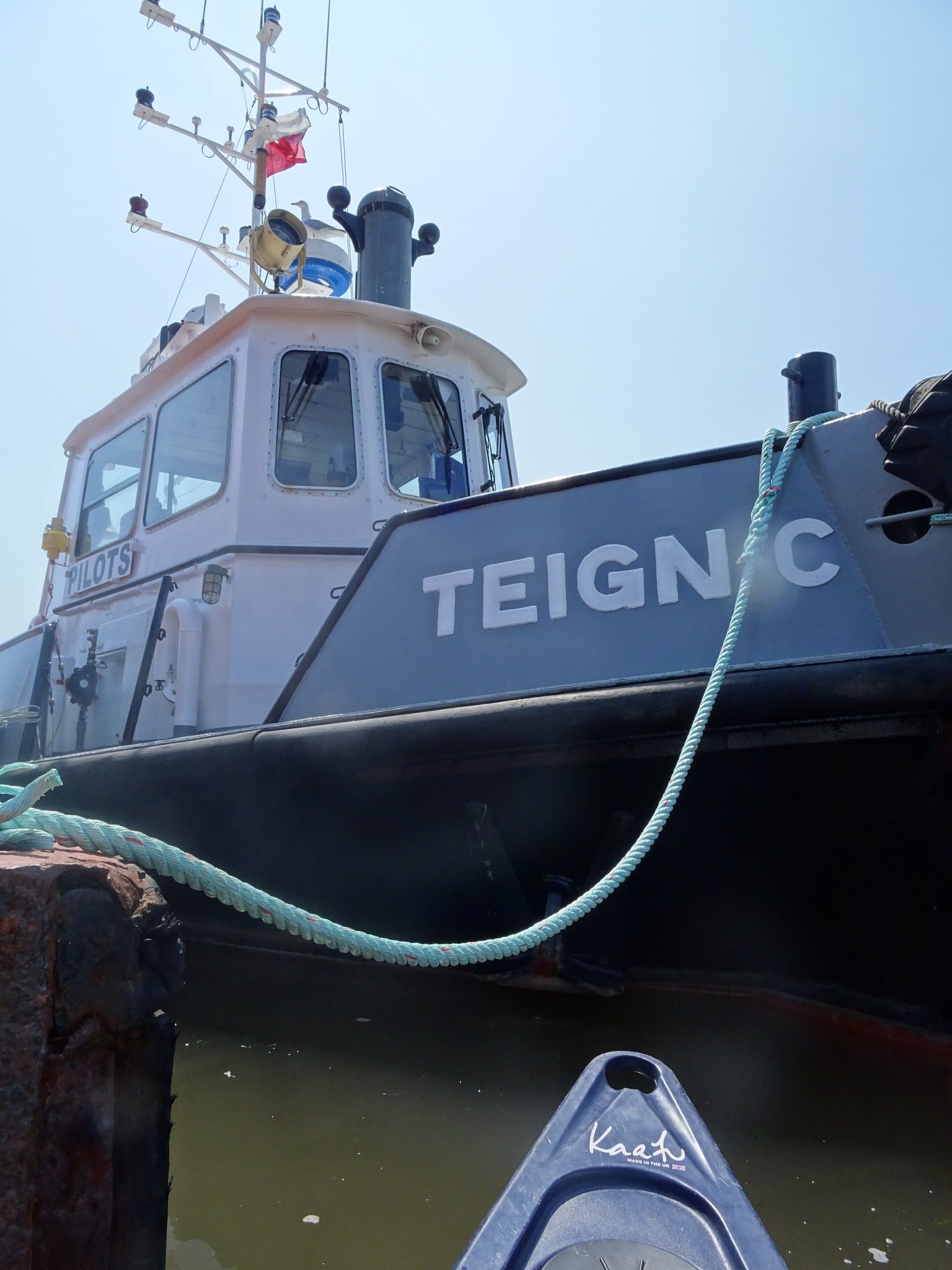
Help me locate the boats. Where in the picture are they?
[454,1051,790,1270]
[0,0,952,1029]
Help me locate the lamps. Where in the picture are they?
[202,564,228,604]
[247,208,308,294]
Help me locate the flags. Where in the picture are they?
[263,107,312,180]
